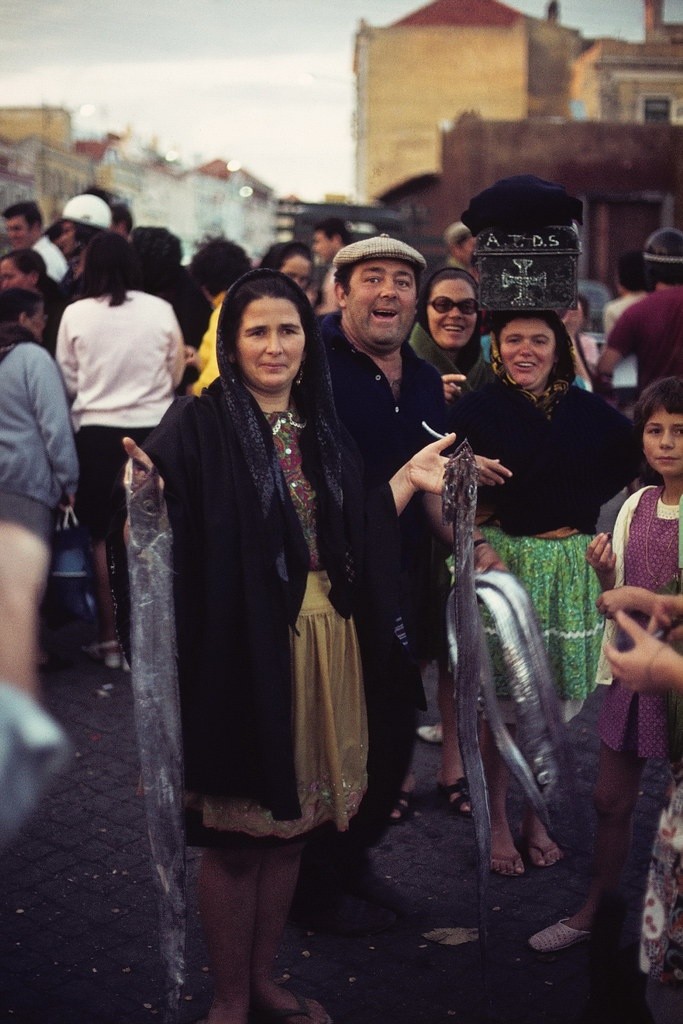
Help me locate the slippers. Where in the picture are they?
[491,838,526,876]
[258,986,332,1024]
[527,918,595,953]
[390,789,413,822]
[437,771,475,817]
[522,821,563,868]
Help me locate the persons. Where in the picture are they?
[528,376,683,952]
[444,222,475,275]
[312,218,350,265]
[123,268,368,1023]
[560,228,683,421]
[422,310,644,876]
[383,267,498,823]
[286,233,511,936]
[0,185,252,703]
[603,609,683,1024]
[261,242,312,290]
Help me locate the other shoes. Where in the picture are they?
[83,639,119,669]
[293,895,378,938]
[39,650,62,674]
[355,874,414,916]
[416,725,443,742]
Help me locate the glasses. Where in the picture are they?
[433,297,477,315]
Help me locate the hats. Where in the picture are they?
[334,234,425,269]
[61,193,112,230]
[641,227,683,263]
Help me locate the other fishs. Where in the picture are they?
[124,432,194,1023]
[445,570,580,855]
[445,437,492,963]
